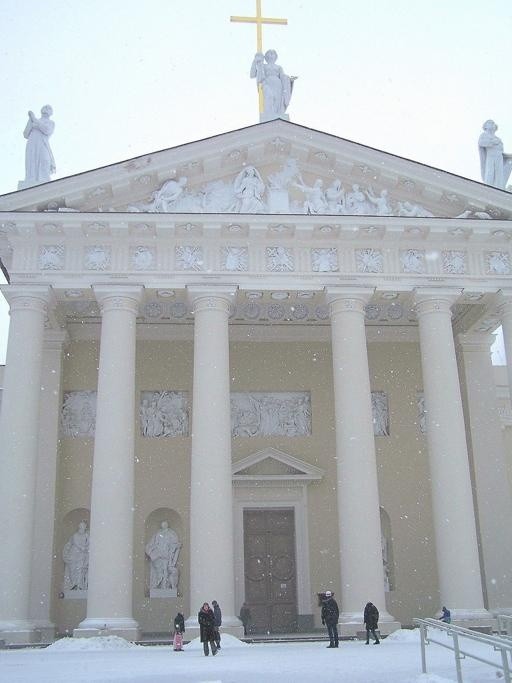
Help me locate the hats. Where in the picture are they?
[326,591,332,597]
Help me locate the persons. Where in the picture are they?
[364,601,380,645]
[152,165,436,218]
[439,606,450,631]
[211,601,222,650]
[62,522,90,591]
[150,522,181,591]
[322,590,339,647]
[24,104,55,186]
[250,49,294,119]
[240,602,251,635]
[140,398,188,437]
[479,120,504,190]
[198,602,218,656]
[174,611,185,650]
[237,396,312,437]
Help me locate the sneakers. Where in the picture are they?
[326,640,380,648]
[173,646,221,656]
